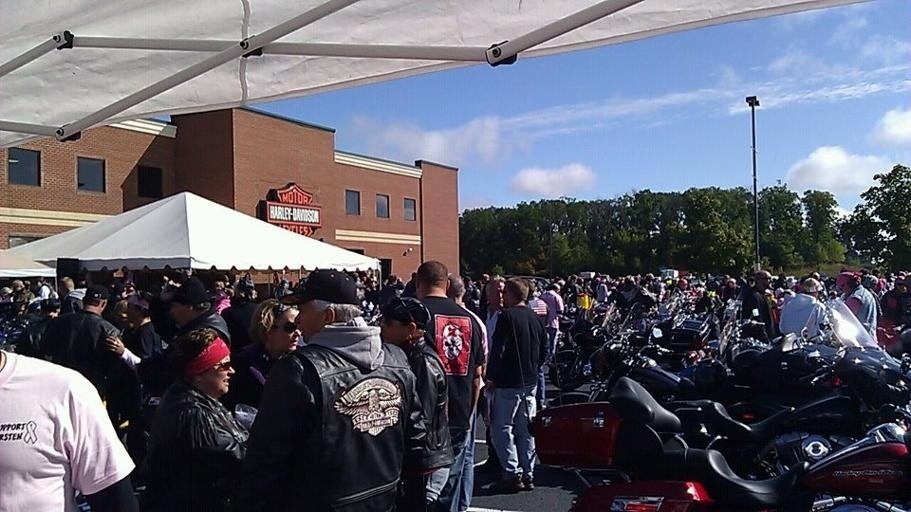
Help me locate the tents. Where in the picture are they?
[2,191,381,277]
[0,250,58,292]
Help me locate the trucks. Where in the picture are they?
[580,270,597,280]
[661,268,680,282]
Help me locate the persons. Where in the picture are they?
[0,271,307,490]
[527,270,911,397]
[485,278,547,489]
[246,270,426,511]
[349,269,412,327]
[417,261,485,511]
[154,329,249,512]
[382,298,455,511]
[464,275,509,476]
[0,351,140,512]
[446,272,489,510]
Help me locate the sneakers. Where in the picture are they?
[474,455,505,476]
[489,477,525,493]
[522,477,535,491]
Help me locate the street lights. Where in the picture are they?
[745,95,765,274]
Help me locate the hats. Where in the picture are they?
[382,296,433,329]
[172,276,211,305]
[802,278,824,292]
[86,282,108,299]
[277,268,360,306]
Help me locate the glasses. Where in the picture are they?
[275,320,299,333]
[209,360,233,370]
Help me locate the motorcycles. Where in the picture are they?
[522,350,910,510]
[509,286,910,473]
